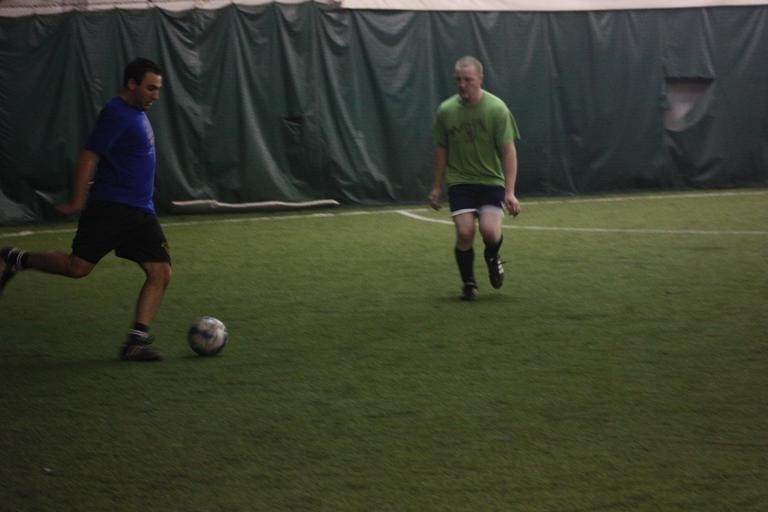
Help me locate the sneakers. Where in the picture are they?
[463,279,479,300]
[485,249,503,288]
[0,247,18,291]
[122,336,164,361]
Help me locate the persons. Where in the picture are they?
[1,56,172,365]
[426,56,521,302]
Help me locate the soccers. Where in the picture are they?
[188,317,228,355]
